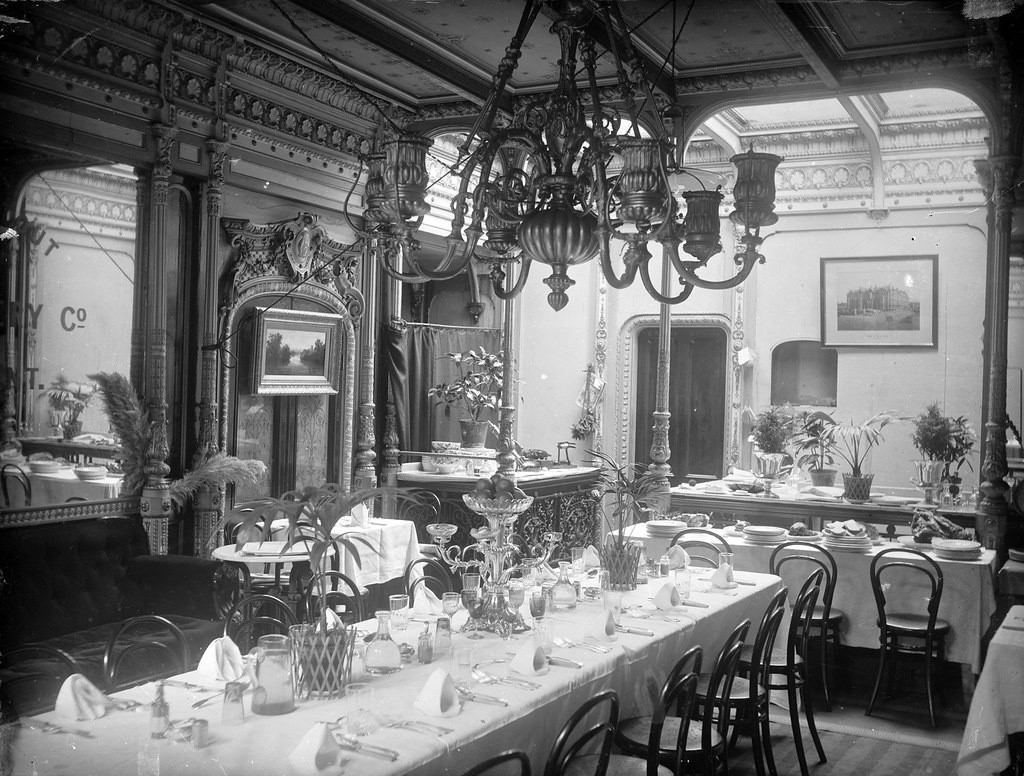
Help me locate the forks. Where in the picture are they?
[319,644,539,765]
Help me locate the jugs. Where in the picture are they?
[246,631,296,714]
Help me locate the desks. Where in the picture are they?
[0,565,800,776]
[350,503,371,526]
[0,460,123,508]
[212,546,338,638]
[234,460,1024,776]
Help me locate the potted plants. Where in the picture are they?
[222,481,446,697]
[745,405,978,507]
[428,347,506,449]
[39,379,96,440]
[580,448,665,584]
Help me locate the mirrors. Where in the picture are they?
[0,148,184,526]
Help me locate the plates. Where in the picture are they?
[421,441,497,475]
[647,516,985,560]
[681,468,976,513]
[30,460,125,480]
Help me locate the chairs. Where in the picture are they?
[0,526,951,776]
[0,463,90,507]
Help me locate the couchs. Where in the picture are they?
[0,520,256,722]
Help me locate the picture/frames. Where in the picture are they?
[250,305,343,397]
[820,257,940,350]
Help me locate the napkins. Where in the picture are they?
[55,674,112,722]
[197,635,245,685]
[709,562,737,589]
[316,609,342,630]
[289,724,345,776]
[415,667,462,716]
[414,585,444,617]
[653,582,686,611]
[590,608,616,642]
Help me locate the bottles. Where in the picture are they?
[362,611,401,674]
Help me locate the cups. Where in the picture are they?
[18,679,244,776]
[390,544,756,656]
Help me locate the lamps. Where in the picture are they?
[343,0,783,312]
